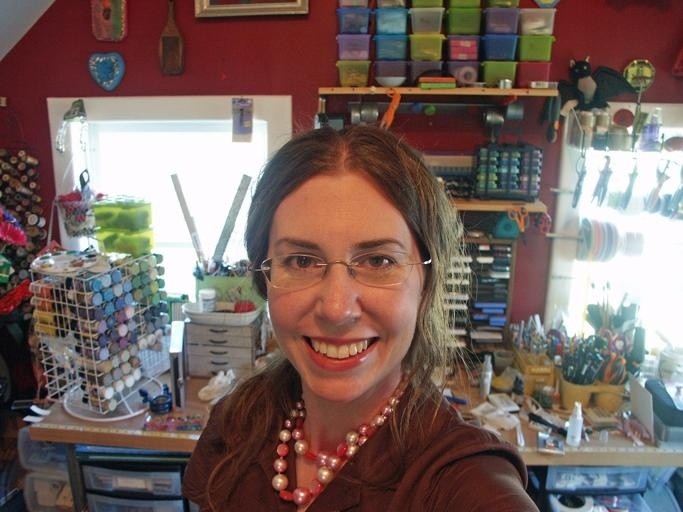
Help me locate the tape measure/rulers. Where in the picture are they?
[169,171,206,266]
[212,171,254,268]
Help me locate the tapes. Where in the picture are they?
[548,494,594,512]
[457,64,477,84]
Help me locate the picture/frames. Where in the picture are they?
[194,0,309,19]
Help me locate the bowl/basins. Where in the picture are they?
[372,76,409,89]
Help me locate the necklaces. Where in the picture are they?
[273,373,411,509]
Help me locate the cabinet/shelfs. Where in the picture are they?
[445,197,547,358]
[317,86,559,213]
[184,311,265,379]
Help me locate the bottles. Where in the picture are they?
[0,141,47,299]
[34,250,171,409]
[564,400,585,447]
[476,352,495,398]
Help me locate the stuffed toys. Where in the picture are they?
[540,54,634,120]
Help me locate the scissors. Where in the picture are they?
[602,329,629,356]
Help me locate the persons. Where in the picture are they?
[179,114,542,511]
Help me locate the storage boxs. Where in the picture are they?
[196,273,266,308]
[16,428,73,511]
[335,0,558,88]
[92,199,154,259]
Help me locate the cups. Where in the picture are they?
[593,382,625,418]
[558,375,598,410]
[196,288,217,314]
[497,77,516,92]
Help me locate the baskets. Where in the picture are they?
[56,199,99,237]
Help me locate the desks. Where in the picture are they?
[28,374,683,512]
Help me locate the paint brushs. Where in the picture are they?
[583,282,629,329]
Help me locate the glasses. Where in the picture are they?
[254,250,432,288]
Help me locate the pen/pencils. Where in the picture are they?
[446,396,466,404]
[547,330,629,384]
[509,313,547,352]
[527,412,566,438]
